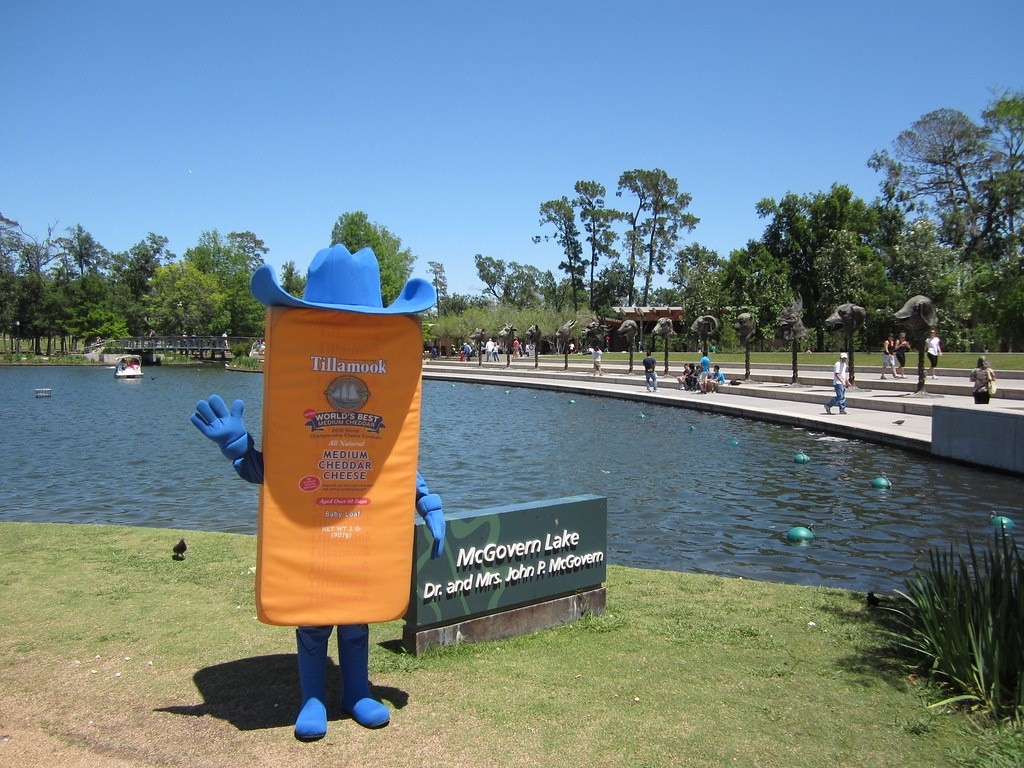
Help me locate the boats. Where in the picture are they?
[113,354,144,379]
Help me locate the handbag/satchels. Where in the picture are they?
[986,368,997,395]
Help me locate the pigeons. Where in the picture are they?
[173,538,187,558]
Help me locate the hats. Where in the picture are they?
[840,352,847,358]
[888,333,895,337]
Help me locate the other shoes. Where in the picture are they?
[932,375,938,380]
[647,387,658,392]
[677,384,716,394]
[881,375,906,380]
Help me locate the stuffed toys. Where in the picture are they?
[190,240,450,753]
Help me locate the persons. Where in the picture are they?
[96,336,102,351]
[592,347,603,376]
[148,329,156,346]
[970,357,996,404]
[221,331,229,349]
[895,333,911,379]
[568,340,593,355]
[824,351,853,414]
[643,351,658,391]
[683,350,725,394]
[182,331,187,346]
[430,336,521,362]
[924,329,943,380]
[880,333,900,379]
[121,359,140,370]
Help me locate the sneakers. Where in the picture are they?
[825,405,831,414]
[840,411,846,414]
